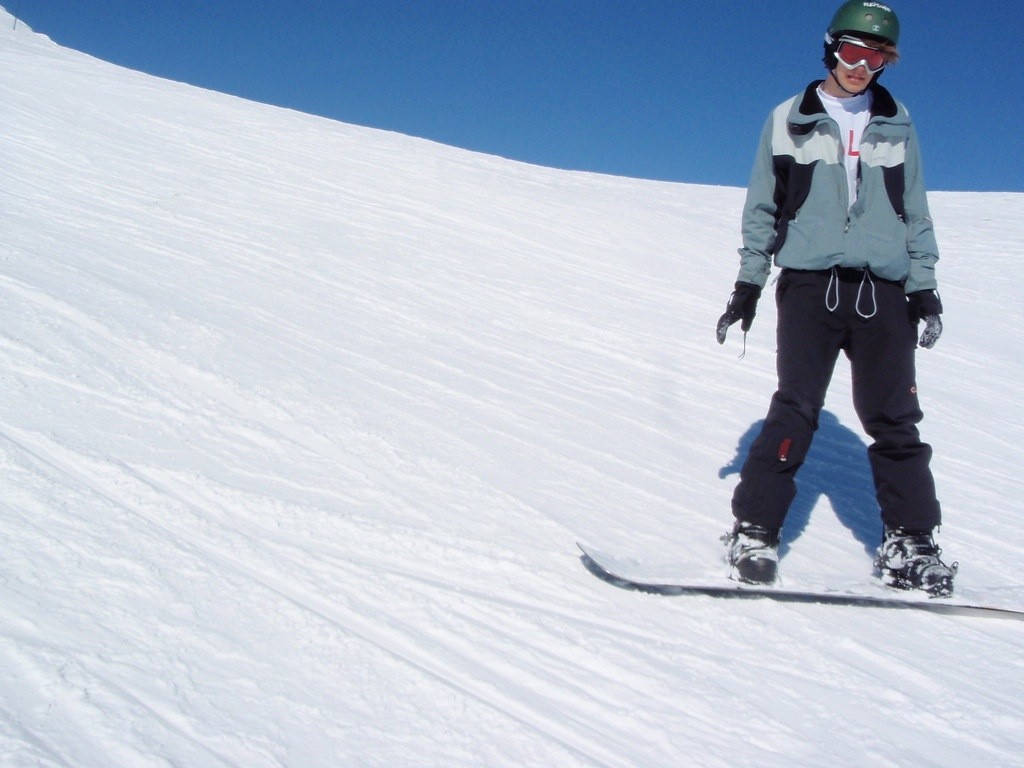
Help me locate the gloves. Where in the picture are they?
[716,280,762,360]
[905,289,943,349]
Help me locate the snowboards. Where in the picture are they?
[568,534,1023,615]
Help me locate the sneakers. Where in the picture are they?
[872,523,959,598]
[718,518,784,588]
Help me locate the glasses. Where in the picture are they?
[832,35,892,75]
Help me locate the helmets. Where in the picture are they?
[823,0,900,44]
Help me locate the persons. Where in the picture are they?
[716,0,960,600]
